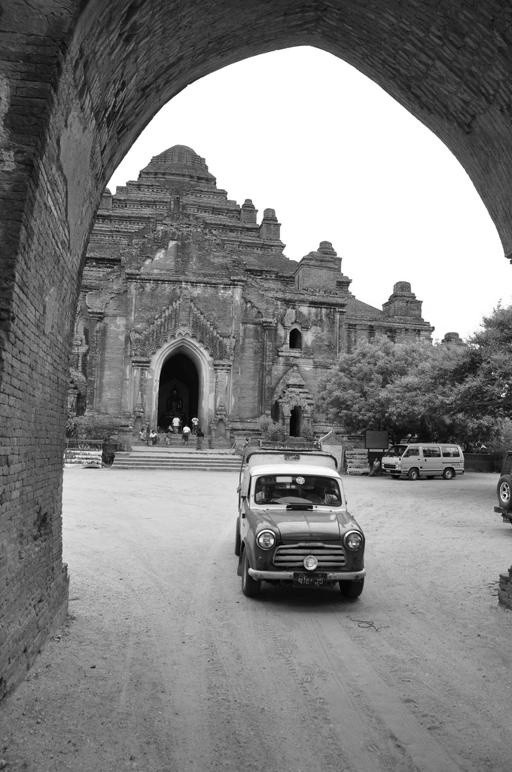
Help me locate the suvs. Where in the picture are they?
[492,447,512,521]
[232,435,366,602]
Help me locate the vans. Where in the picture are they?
[380,441,467,481]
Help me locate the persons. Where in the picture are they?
[256,479,280,505]
[368,458,380,477]
[478,442,486,450]
[307,481,338,506]
[139,416,204,450]
[464,440,473,453]
